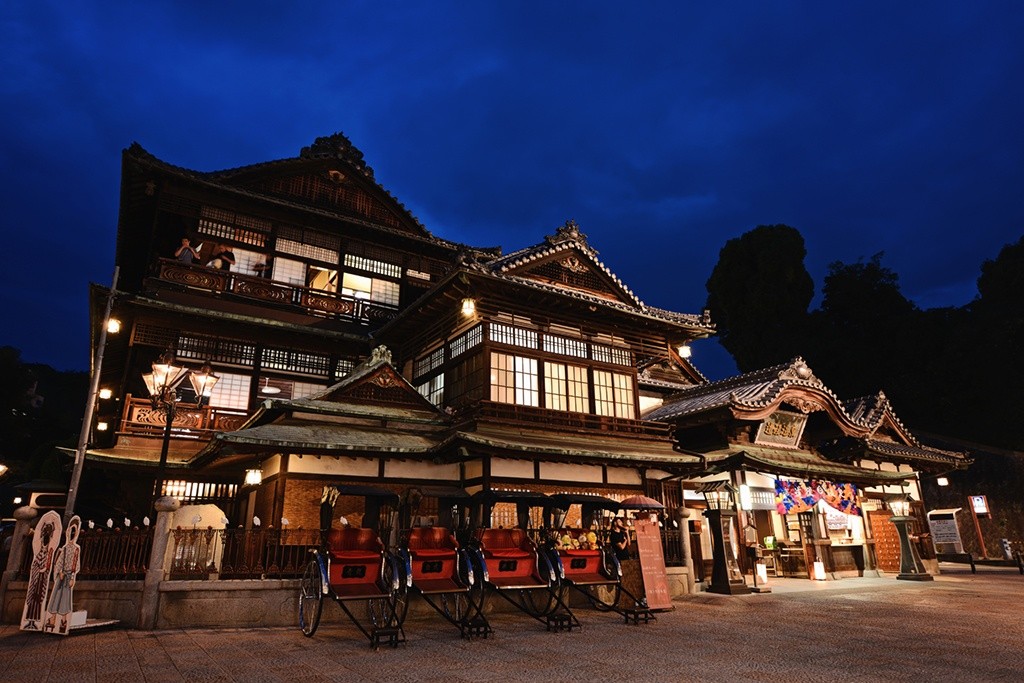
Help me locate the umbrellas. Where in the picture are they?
[618,496,668,513]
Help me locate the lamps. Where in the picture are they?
[936,475,949,486]
[756,558,768,585]
[695,480,739,510]
[884,493,915,517]
[677,341,692,358]
[460,283,477,316]
[243,453,264,486]
[814,557,827,580]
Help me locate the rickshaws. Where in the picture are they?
[297,483,657,650]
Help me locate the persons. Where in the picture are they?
[174,237,200,264]
[609,517,631,560]
[207,243,236,271]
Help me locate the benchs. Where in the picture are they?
[471,527,535,577]
[545,528,603,573]
[328,526,384,585]
[407,525,457,580]
